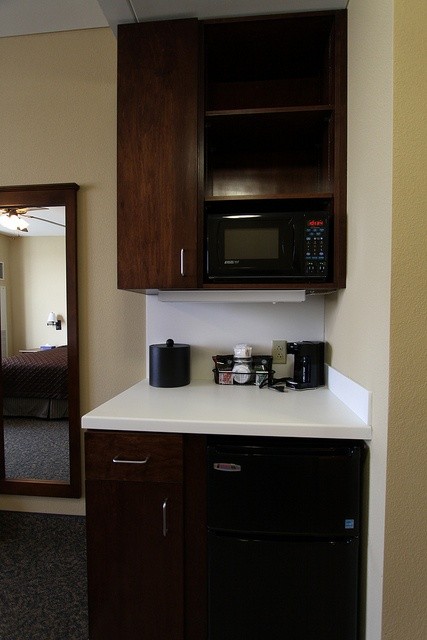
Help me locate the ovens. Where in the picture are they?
[207,431,369,640]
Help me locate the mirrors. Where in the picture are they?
[0,182,81,500]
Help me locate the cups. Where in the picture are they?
[255,371,268,386]
[219,370,233,385]
[232,345,252,359]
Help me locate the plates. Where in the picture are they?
[231,364,251,384]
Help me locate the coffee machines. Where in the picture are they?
[286,341,326,389]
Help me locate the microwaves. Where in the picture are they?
[203,210,330,279]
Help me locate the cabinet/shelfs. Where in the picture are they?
[115,17,201,295]
[201,8,348,293]
[83,429,207,640]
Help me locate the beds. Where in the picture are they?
[0,344,68,420]
